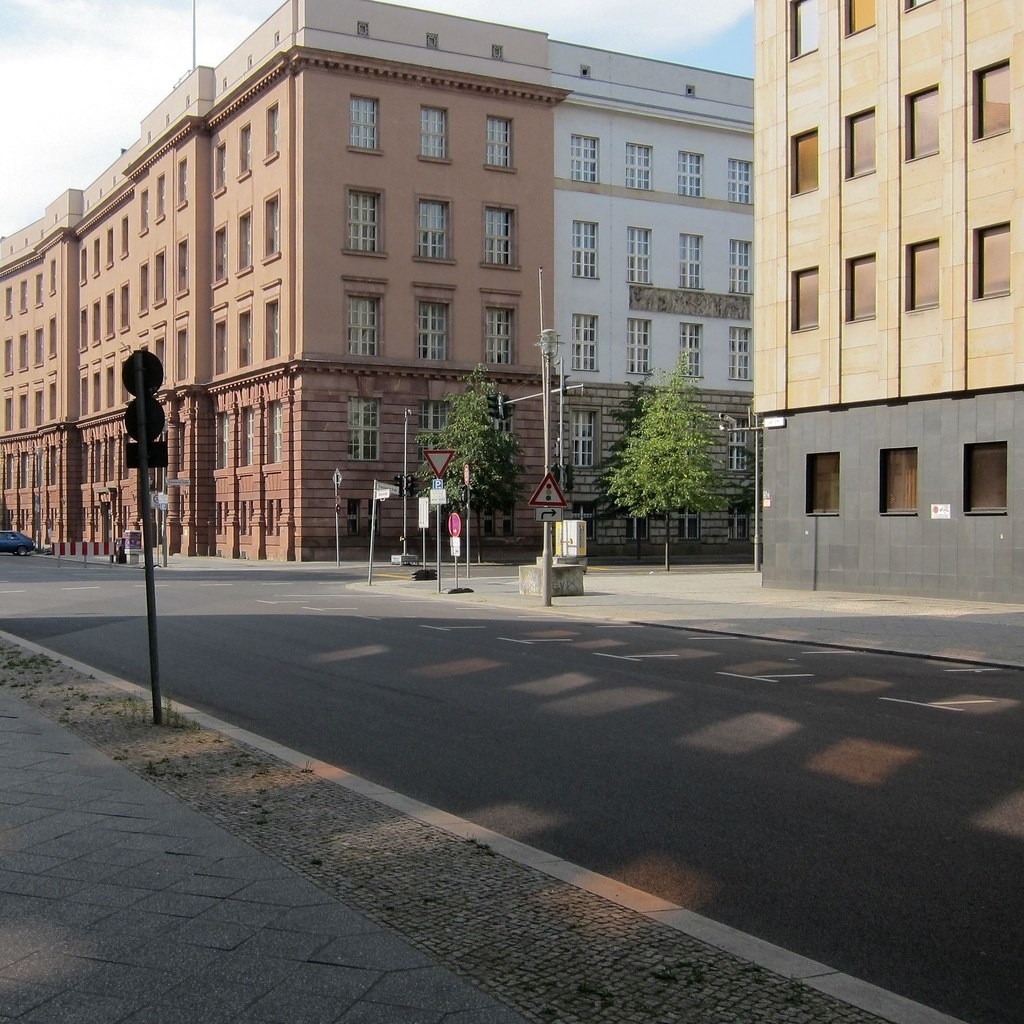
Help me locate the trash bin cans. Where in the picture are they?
[115,538,126,564]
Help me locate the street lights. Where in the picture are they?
[35,446,43,551]
[535,329,561,610]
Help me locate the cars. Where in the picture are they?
[0,529,35,556]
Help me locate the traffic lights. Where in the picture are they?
[502,394,513,419]
[486,394,499,419]
[406,475,418,498]
[393,474,404,498]
